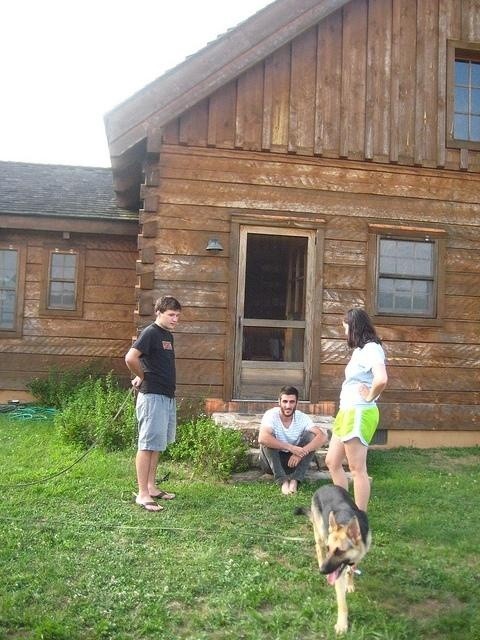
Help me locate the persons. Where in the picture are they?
[325,309,386,513]
[125,294,182,512]
[259,385,327,495]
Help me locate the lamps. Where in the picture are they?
[206,236,224,256]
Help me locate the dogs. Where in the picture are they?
[293,484,372,636]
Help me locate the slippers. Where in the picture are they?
[137,498,163,512]
[152,491,174,499]
